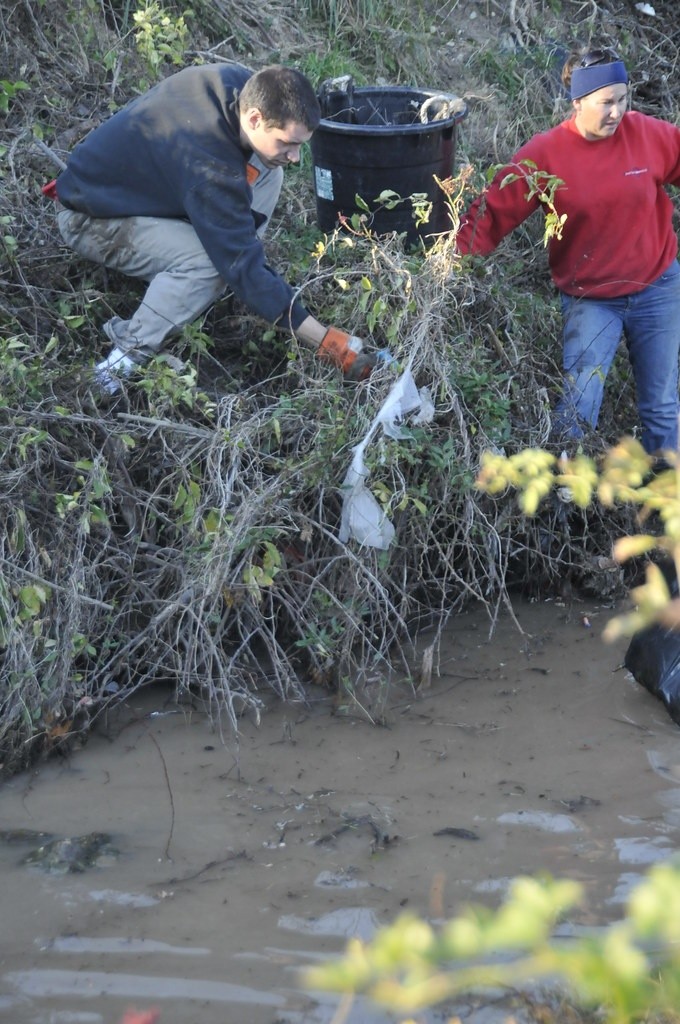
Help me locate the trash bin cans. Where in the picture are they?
[311,86,469,244]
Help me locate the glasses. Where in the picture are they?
[581,48,621,67]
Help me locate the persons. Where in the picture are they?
[440,45,680,485]
[49,63,378,402]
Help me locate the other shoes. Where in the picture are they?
[94,350,140,400]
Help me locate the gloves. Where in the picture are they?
[319,327,373,381]
